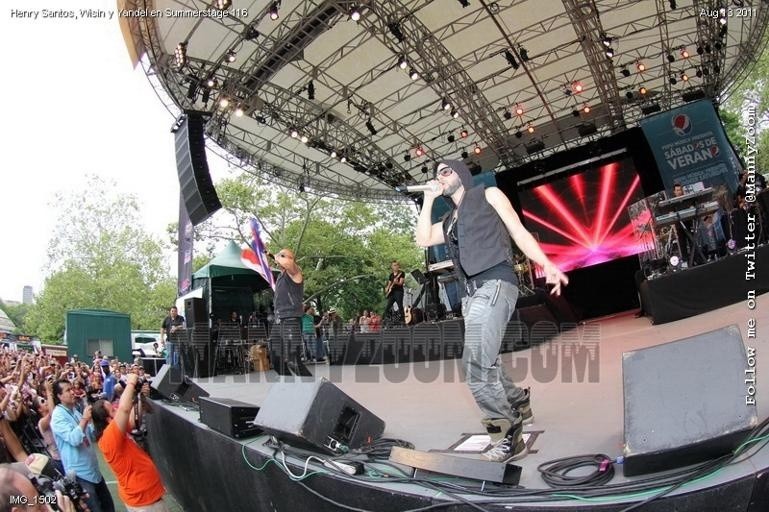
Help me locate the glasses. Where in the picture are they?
[40,398,46,404]
[440,167,453,176]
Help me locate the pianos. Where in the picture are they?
[657,187,713,216]
[654,201,720,229]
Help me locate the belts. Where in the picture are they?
[463,279,481,296]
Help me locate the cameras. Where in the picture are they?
[134,376,146,393]
[27,473,85,512]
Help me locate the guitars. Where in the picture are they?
[385,272,402,298]
[403,288,418,325]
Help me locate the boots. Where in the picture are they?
[513,386,535,425]
[480,411,530,464]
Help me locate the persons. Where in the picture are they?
[414,159,571,469]
[268,248,313,378]
[301,302,380,364]
[0,343,170,512]
[161,306,185,376]
[672,167,766,269]
[379,261,407,327]
[209,309,272,376]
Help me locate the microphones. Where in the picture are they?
[659,194,663,201]
[394,184,432,192]
[263,250,275,260]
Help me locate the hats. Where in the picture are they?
[101,360,109,366]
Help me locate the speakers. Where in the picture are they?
[150,363,210,404]
[253,375,386,458]
[198,396,264,439]
[621,323,760,477]
[425,303,448,322]
[174,113,222,227]
[184,298,209,328]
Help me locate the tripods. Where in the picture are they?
[515,258,537,296]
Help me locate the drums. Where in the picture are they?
[513,264,524,273]
[521,262,528,273]
